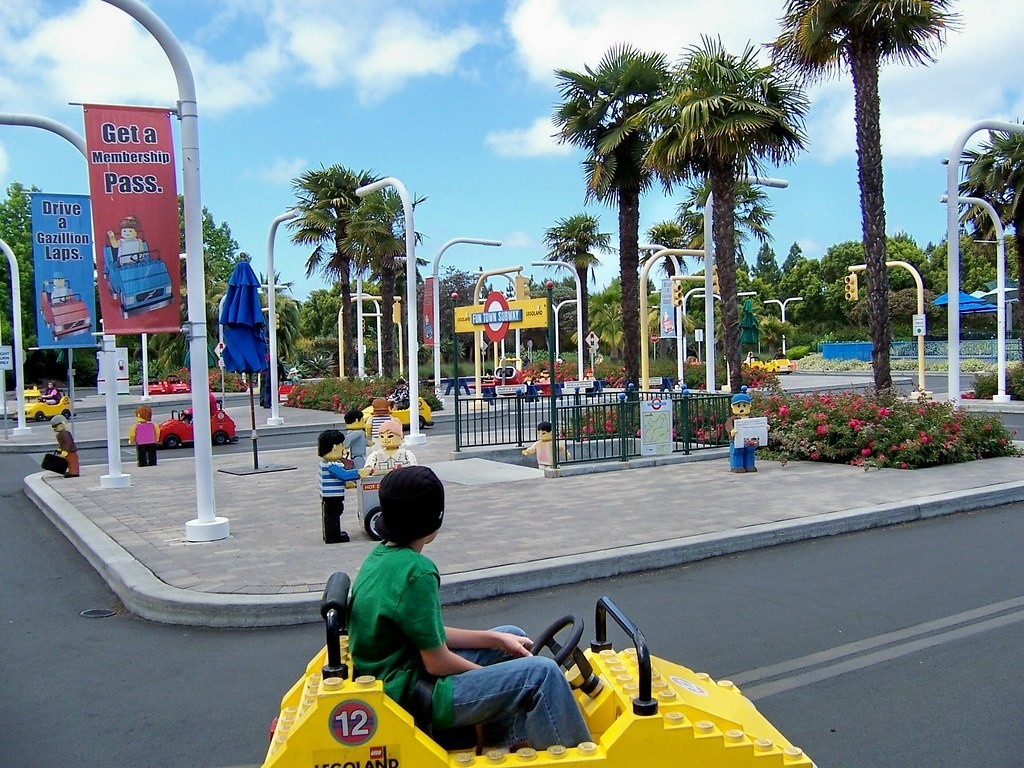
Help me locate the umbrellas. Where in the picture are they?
[931,290,998,315]
[741,300,759,351]
[220,252,267,471]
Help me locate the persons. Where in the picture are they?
[485,369,493,380]
[41,381,60,405]
[366,399,394,439]
[182,382,218,422]
[745,351,754,362]
[51,415,79,477]
[774,347,785,360]
[724,394,770,473]
[522,356,530,366]
[363,418,418,475]
[129,406,161,466]
[522,422,571,469]
[259,339,285,408]
[317,430,370,544]
[343,410,374,470]
[347,466,592,751]
[388,378,410,411]
[558,356,562,363]
[583,370,596,381]
[288,367,300,385]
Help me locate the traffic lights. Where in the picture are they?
[712,264,721,295]
[361,317,366,332]
[275,312,280,330]
[844,274,859,301]
[672,282,683,307]
[515,276,530,300]
[390,302,401,323]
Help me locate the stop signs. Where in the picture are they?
[651,334,659,343]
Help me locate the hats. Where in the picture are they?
[375,465,444,543]
